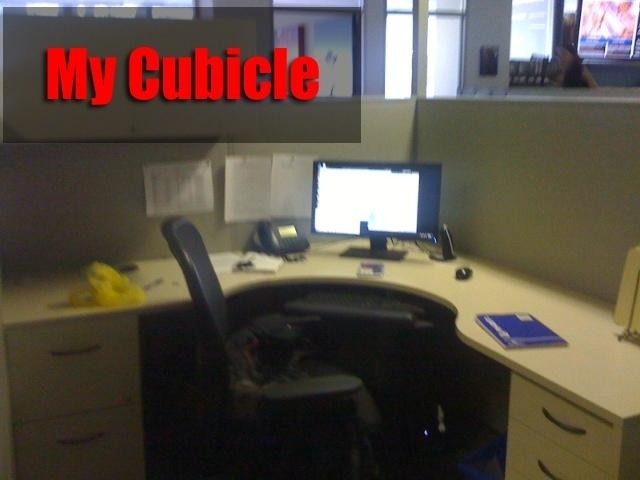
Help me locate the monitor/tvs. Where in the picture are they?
[310,159,443,260]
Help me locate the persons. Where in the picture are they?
[543,39,602,88]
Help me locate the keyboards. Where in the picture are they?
[281,287,429,327]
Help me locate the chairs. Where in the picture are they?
[158,212,438,479]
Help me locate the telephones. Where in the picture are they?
[256,218,310,256]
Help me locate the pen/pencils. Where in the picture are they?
[143,278,164,291]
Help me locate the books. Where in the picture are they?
[234,248,288,274]
[474,310,569,351]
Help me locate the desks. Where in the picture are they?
[0,240,640,478]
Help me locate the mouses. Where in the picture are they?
[456,266,472,280]
